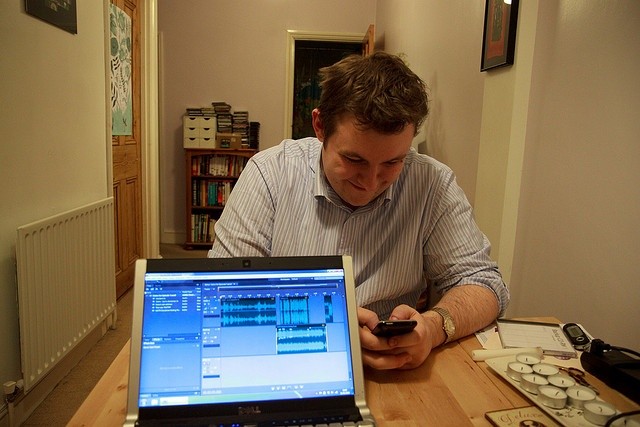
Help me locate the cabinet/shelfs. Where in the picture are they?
[183,146,259,248]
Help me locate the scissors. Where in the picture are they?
[559,366,600,397]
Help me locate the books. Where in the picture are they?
[190,213,217,242]
[232,111,250,148]
[192,154,247,177]
[212,101,233,132]
[192,179,233,206]
[186,107,217,117]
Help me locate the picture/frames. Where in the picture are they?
[22,1,77,35]
[480,2,519,73]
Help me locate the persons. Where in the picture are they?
[206,50,512,371]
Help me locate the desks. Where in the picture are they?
[64,317,640,426]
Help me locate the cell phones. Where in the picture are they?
[372,319,419,336]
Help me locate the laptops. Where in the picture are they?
[121,256,373,427]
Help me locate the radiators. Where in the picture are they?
[3,195,115,398]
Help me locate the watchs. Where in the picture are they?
[429,305,457,346]
